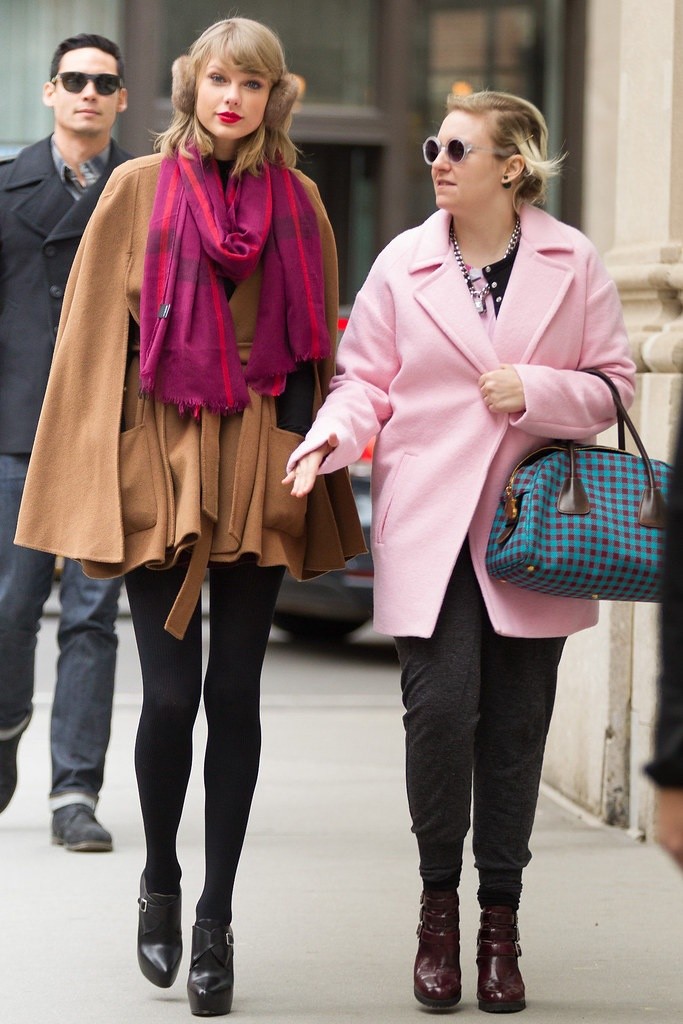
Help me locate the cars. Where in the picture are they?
[262,307,373,644]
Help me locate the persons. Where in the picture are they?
[1,35,146,851]
[280,93,641,1015]
[11,18,368,1018]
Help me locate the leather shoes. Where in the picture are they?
[51,803,113,851]
[0,704,32,812]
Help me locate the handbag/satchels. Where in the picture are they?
[485,370,674,603]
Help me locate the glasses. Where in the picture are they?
[422,136,514,167]
[51,71,124,96]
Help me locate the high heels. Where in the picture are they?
[413,888,461,1008]
[476,905,525,1011]
[187,919,234,1014]
[137,868,182,988]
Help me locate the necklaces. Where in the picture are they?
[446,222,522,310]
